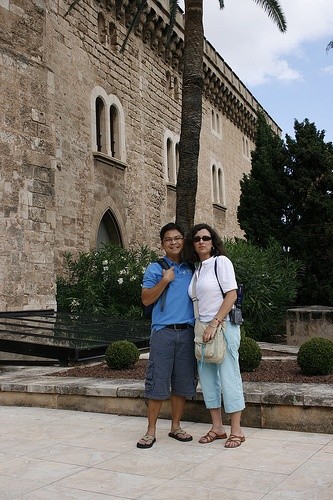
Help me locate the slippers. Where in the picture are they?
[169,428,193,441]
[198,430,228,444]
[224,435,245,448]
[137,435,156,448]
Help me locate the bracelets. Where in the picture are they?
[214,317,222,323]
[209,323,216,328]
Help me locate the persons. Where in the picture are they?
[185,224,246,448]
[136,222,196,448]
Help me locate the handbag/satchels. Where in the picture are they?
[193,319,227,364]
[230,308,243,323]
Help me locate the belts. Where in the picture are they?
[166,324,190,331]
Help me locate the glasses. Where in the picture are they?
[162,237,183,242]
[193,236,211,242]
